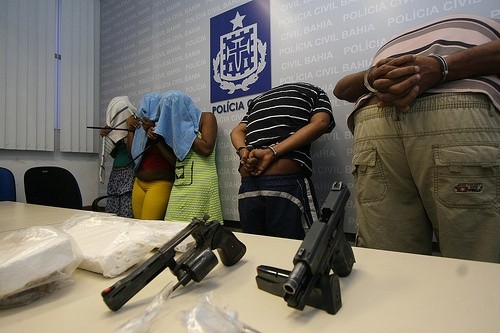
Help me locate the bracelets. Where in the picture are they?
[427,53,448,90]
[262,146,276,161]
[236,145,252,157]
[364,66,378,93]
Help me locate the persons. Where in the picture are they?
[147,91,223,225]
[229,82,336,240]
[100,95,138,217]
[332,13,500,264]
[127,92,174,220]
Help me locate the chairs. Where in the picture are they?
[0,166,16,202]
[23,165,109,212]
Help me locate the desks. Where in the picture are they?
[0,203,500,332]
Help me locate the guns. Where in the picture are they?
[101,214,247,312]
[256,181,356,316]
